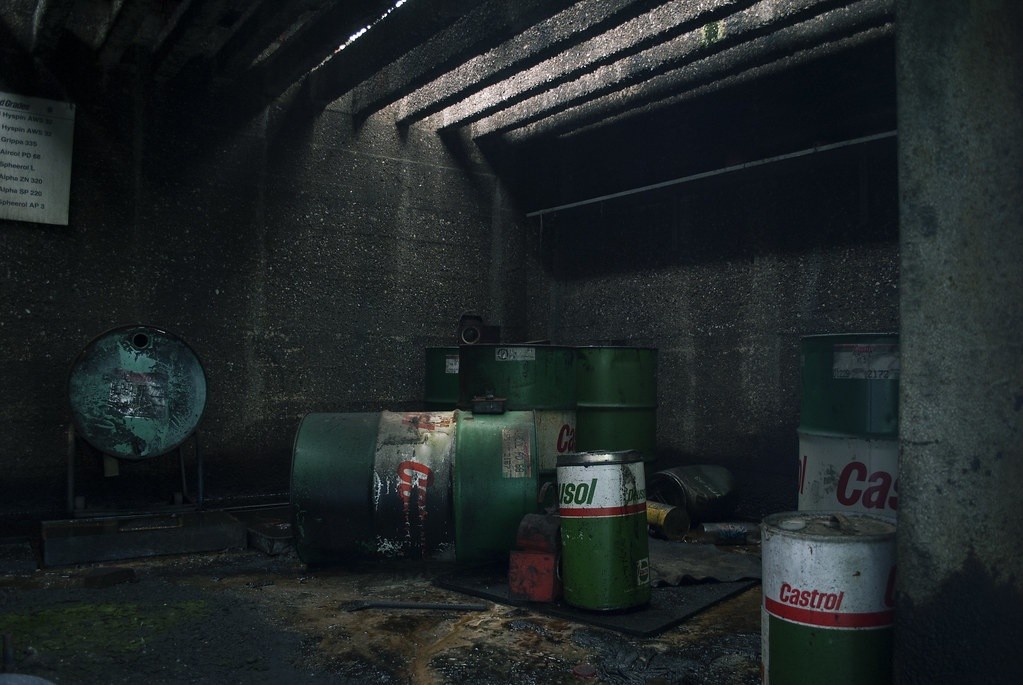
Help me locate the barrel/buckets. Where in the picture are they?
[421,340,660,507]
[798,332,901,515]
[646,499,692,542]
[65,323,208,460]
[555,449,651,611]
[646,464,735,525]
[760,510,896,685]
[289,412,539,569]
